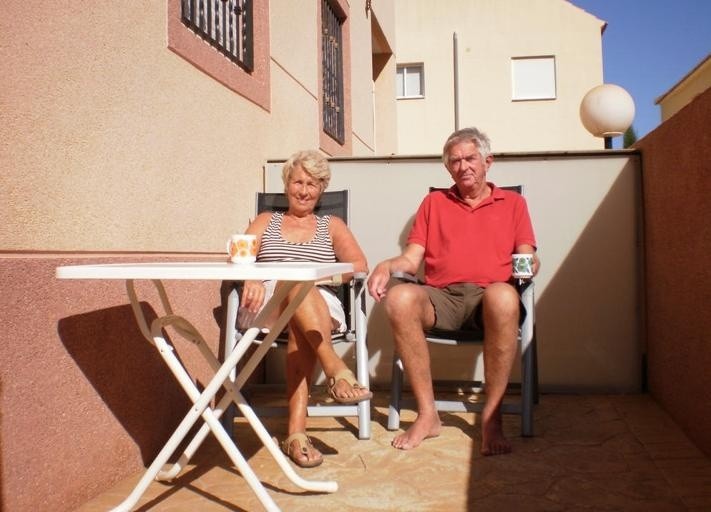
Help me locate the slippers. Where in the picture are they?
[281,433,324,468]
[328,369,373,404]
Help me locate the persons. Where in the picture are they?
[240,149,373,467]
[368,128,540,456]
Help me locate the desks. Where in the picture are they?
[54,258,353,510]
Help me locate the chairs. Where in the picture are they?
[224,185,372,439]
[387,185,539,440]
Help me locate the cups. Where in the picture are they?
[512,254,535,279]
[227,235,258,264]
